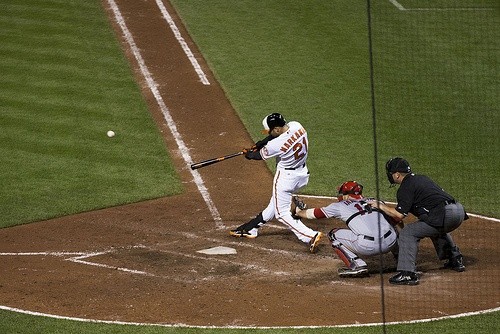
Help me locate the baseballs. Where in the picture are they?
[107,130,115,138]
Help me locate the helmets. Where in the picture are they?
[338,181,363,195]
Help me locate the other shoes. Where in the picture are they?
[230,223,259,238]
[309,232,324,253]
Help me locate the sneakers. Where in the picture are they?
[389,270,420,285]
[336,266,369,278]
[443,254,465,272]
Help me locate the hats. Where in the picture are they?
[259,113,287,135]
[385,157,411,173]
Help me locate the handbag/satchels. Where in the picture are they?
[421,207,445,228]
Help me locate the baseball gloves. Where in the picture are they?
[290,196,308,220]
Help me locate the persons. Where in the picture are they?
[291,181,399,276]
[367,157,469,286]
[230,112,322,254]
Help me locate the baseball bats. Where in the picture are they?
[190,147,255,170]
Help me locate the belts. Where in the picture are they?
[285,162,306,171]
[364,230,391,241]
[441,199,455,206]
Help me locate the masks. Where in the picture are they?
[387,172,395,184]
